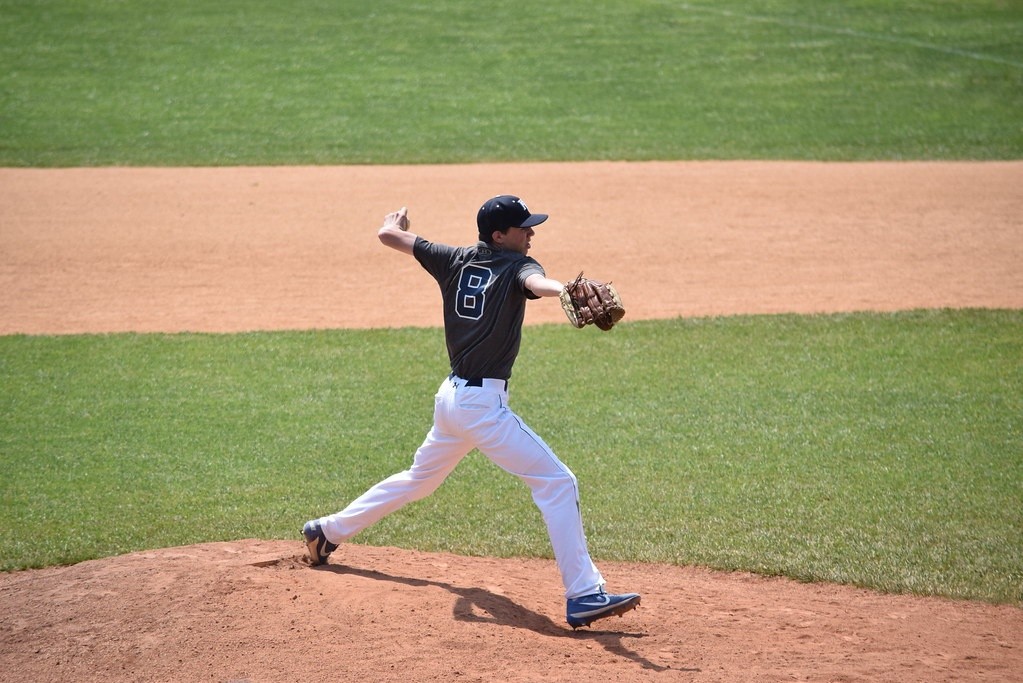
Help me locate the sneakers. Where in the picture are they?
[300,519,340,566]
[566,593,641,631]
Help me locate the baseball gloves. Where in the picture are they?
[560,273,626,332]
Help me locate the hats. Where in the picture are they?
[477,195,548,236]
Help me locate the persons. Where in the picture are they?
[300,193,643,629]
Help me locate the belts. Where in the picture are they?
[448,372,507,392]
